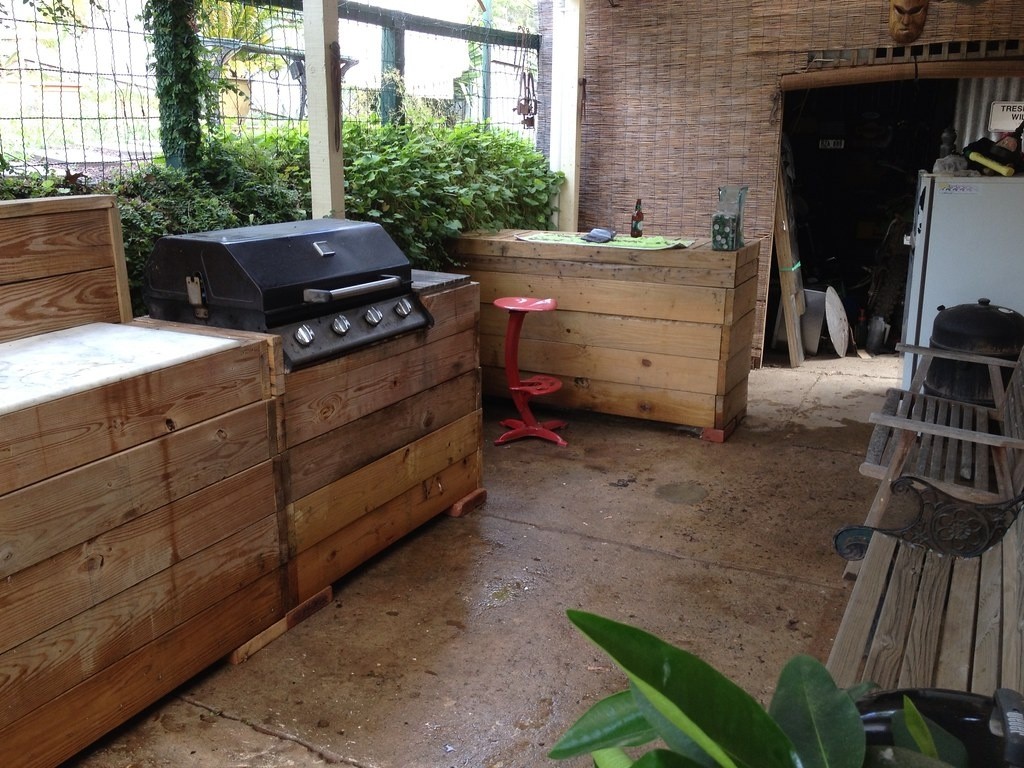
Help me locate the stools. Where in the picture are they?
[492,294,568,448]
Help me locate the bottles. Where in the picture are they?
[631,198,645,237]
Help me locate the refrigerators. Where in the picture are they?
[899,169,1024,399]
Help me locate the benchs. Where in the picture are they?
[821,473,1024,767]
[837,342,1024,583]
